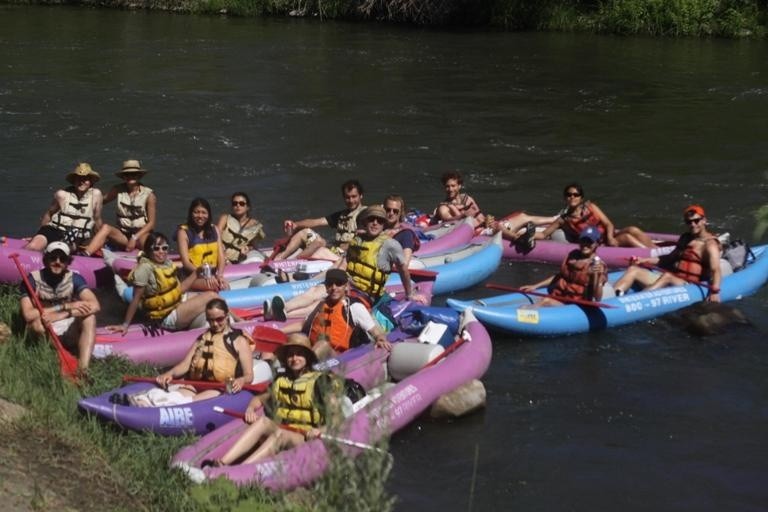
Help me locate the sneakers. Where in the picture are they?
[264,294,288,322]
[523,222,535,249]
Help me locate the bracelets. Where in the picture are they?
[710,290,719,295]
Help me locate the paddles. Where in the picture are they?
[8,252,85,384]
[624,255,720,292]
[253,325,289,355]
[296,255,438,282]
[260,237,288,267]
[486,281,620,309]
[214,405,393,483]
[122,374,272,393]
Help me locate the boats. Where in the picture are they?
[168,304,493,498]
[494,214,733,270]
[0,233,186,291]
[437,243,766,336]
[75,295,426,437]
[68,276,436,368]
[113,230,504,321]
[98,207,482,286]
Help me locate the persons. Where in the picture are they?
[532,184,656,248]
[520,226,609,312]
[437,171,563,241]
[613,205,721,305]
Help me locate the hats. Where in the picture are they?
[44,241,71,256]
[684,206,704,217]
[275,333,318,365]
[115,159,147,179]
[579,226,600,242]
[326,268,349,280]
[359,205,392,228]
[67,163,100,183]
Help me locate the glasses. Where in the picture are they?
[384,208,400,214]
[566,192,581,197]
[367,216,385,225]
[232,200,247,206]
[206,314,226,322]
[326,280,344,287]
[686,217,704,225]
[150,245,169,252]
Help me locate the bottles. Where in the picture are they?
[225,376,234,395]
[202,261,211,279]
